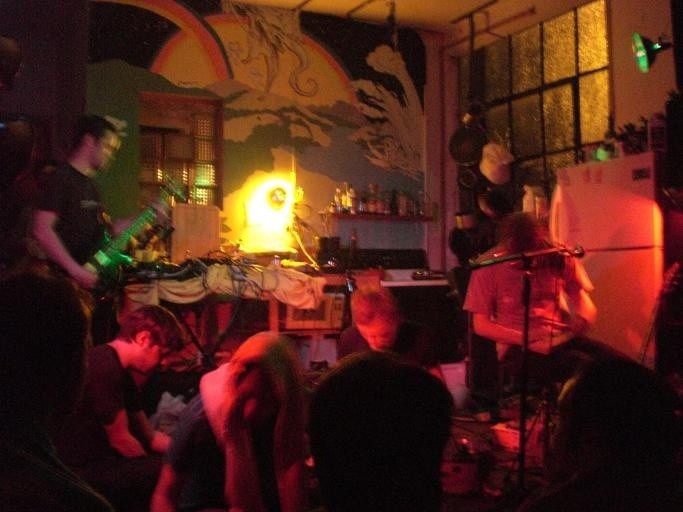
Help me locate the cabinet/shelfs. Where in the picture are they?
[137,92,224,261]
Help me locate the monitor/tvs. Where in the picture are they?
[285,292,345,329]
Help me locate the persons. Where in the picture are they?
[28,111,169,344]
[459,208,599,424]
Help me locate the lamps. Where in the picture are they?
[630,33,673,75]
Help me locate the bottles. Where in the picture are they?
[329,181,425,217]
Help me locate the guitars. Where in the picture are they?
[53,173,188,306]
